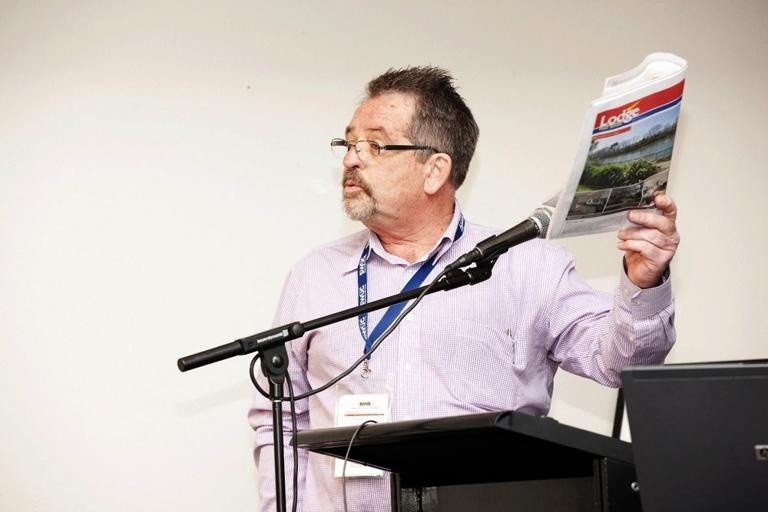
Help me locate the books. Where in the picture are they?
[548,51,689,240]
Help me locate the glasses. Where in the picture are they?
[330,137,439,158]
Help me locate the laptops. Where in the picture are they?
[619,358,767,511]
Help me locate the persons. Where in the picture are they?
[248,62,678,511]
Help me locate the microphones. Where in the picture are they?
[448,206,555,269]
